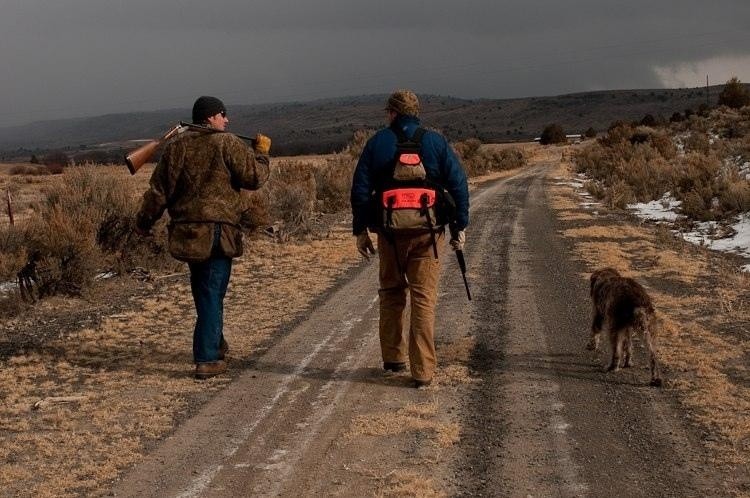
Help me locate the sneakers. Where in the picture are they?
[383,362,406,373]
[412,378,433,390]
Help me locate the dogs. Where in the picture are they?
[586,267,663,387]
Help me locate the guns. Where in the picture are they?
[444,189,472,301]
[125,122,256,175]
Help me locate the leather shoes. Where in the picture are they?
[194,339,228,379]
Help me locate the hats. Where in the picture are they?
[191,95,227,125]
[379,89,420,120]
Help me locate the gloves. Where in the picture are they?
[449,229,466,252]
[251,133,273,158]
[134,225,154,237]
[355,230,377,259]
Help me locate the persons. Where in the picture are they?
[350,86,469,390]
[134,95,273,379]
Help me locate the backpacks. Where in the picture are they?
[374,126,439,235]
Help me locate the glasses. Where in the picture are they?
[219,111,227,118]
